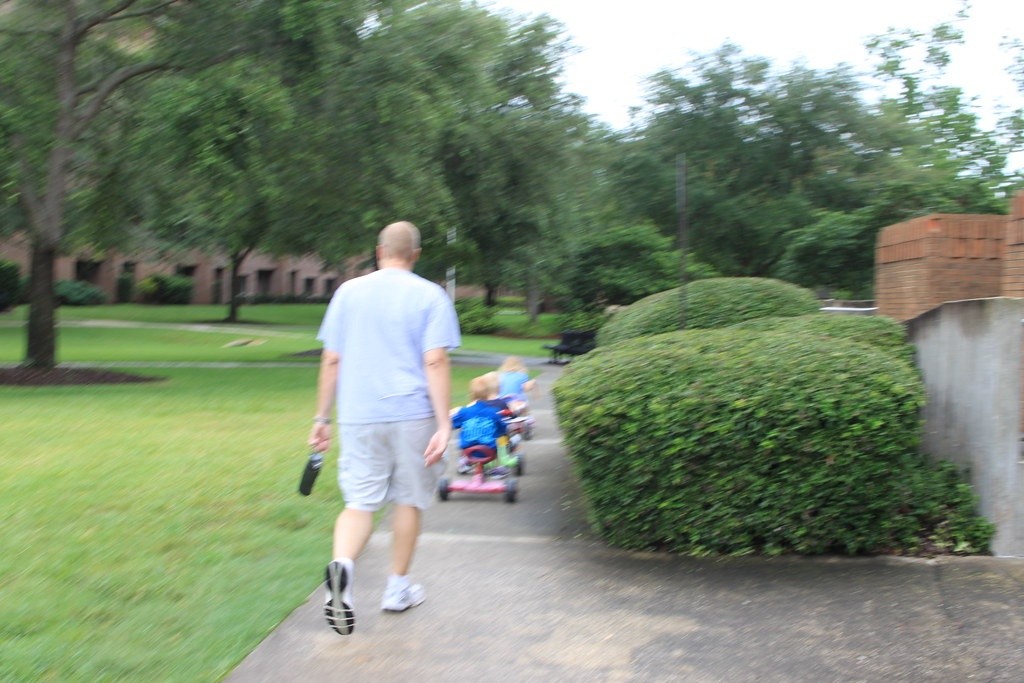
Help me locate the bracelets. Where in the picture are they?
[311,415,330,423]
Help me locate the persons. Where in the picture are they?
[307,220,460,635]
[450,355,537,477]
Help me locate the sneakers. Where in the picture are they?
[381,574,426,610]
[324,556,357,635]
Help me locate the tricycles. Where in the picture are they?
[438,443,525,503]
